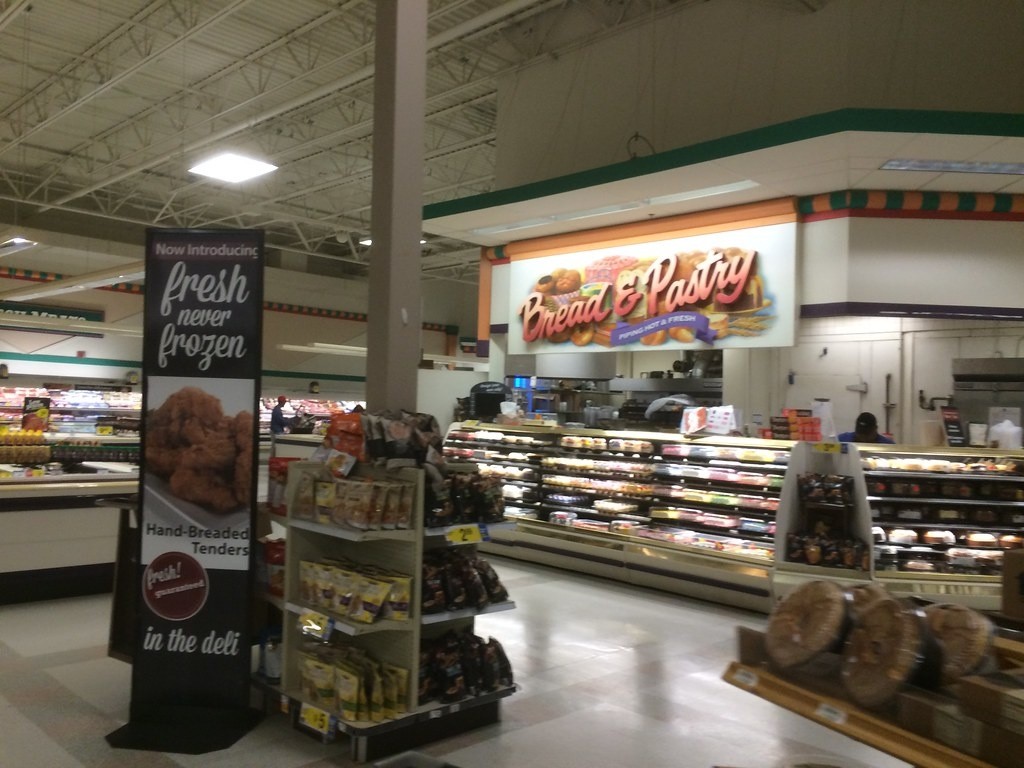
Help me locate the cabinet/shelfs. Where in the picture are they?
[255,456,520,759]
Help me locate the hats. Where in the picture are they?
[278,396,286,403]
[855,411,877,433]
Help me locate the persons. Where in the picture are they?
[271,396,292,433]
[838,413,896,444]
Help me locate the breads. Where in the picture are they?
[763,579,991,706]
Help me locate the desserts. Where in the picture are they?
[532,248,761,347]
